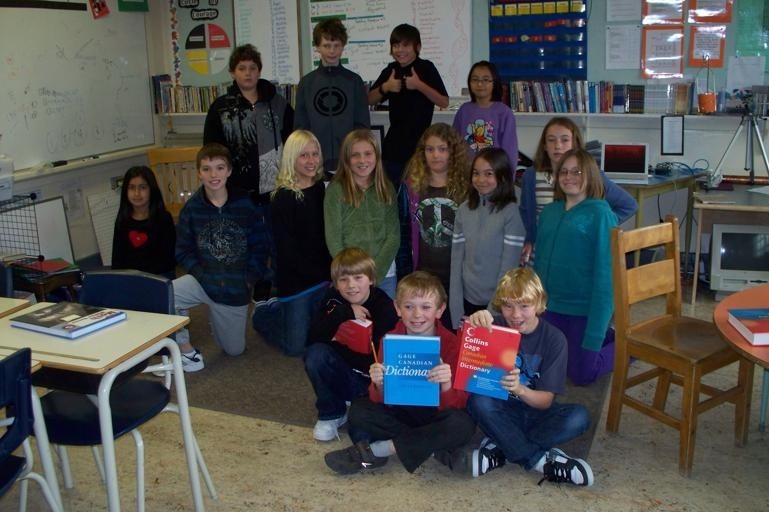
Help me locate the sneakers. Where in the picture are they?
[268,298,280,311]
[433,446,473,472]
[326,440,387,472]
[544,449,595,486]
[473,436,506,476]
[254,302,267,315]
[254,278,272,303]
[153,352,203,376]
[315,413,348,440]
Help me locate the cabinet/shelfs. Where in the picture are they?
[160,110,769,122]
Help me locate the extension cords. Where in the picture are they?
[654,162,671,175]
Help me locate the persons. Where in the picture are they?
[294,19,372,186]
[203,45,295,193]
[468,266,594,487]
[111,166,176,275]
[451,60,517,182]
[518,116,638,266]
[252,130,331,356]
[324,128,401,289]
[368,23,449,184]
[403,123,471,329]
[304,247,400,441]
[152,143,272,377]
[450,147,527,334]
[324,272,471,476]
[532,148,638,387]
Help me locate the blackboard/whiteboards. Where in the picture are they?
[0,1,161,182]
[232,0,302,87]
[307,0,473,100]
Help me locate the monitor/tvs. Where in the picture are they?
[600,142,648,184]
[710,224,769,301]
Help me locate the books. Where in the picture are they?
[9,300,126,340]
[332,317,374,369]
[727,307,769,347]
[452,319,521,400]
[269,80,298,111]
[383,334,440,406]
[11,256,81,283]
[152,73,232,114]
[500,80,698,113]
[0,250,26,262]
[696,193,735,203]
[752,84,769,115]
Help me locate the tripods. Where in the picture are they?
[709,114,768,186]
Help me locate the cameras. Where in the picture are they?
[735,95,755,114]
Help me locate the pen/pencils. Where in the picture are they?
[371,341,378,363]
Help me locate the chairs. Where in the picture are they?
[606,214,755,478]
[0,346,59,512]
[148,148,201,224]
[0,265,14,297]
[29,273,217,512]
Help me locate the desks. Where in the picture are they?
[0,301,207,512]
[601,168,711,281]
[691,190,769,303]
[714,282,769,432]
[0,297,31,318]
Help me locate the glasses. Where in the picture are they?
[560,170,583,176]
[469,78,496,84]
